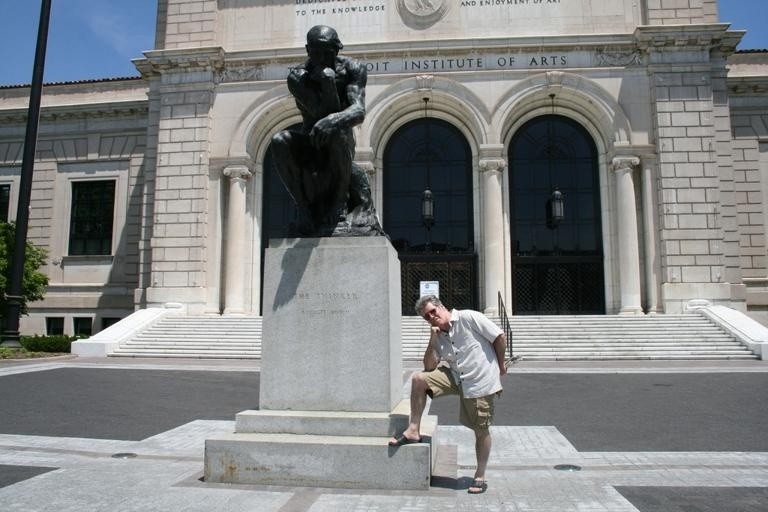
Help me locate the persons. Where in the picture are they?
[386,294,509,496]
[269,23,382,238]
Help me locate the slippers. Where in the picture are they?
[468,480,488,493]
[390,433,422,446]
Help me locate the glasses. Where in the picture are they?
[423,307,438,320]
